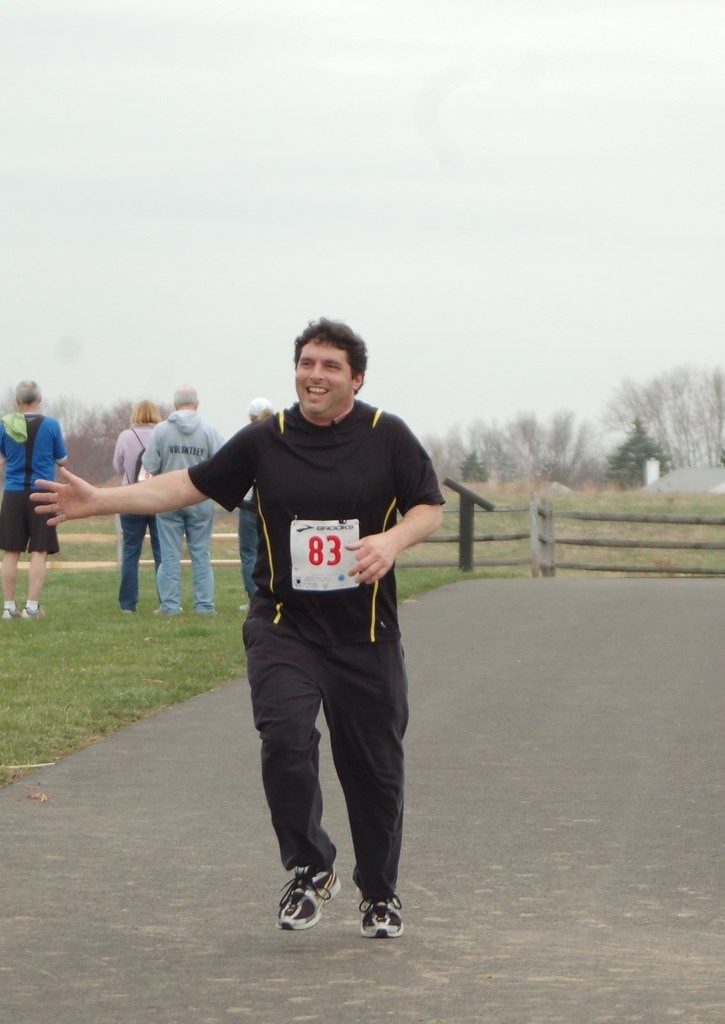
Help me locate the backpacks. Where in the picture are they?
[125,428,157,486]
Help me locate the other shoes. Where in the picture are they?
[120,609,133,614]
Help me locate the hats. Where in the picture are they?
[247,396,276,417]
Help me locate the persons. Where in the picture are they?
[28,317,447,941]
[0,379,68,619]
[112,384,275,616]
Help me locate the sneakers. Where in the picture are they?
[1,605,21,620]
[238,603,251,612]
[358,893,405,938]
[157,609,182,616]
[276,862,342,931]
[21,604,48,619]
